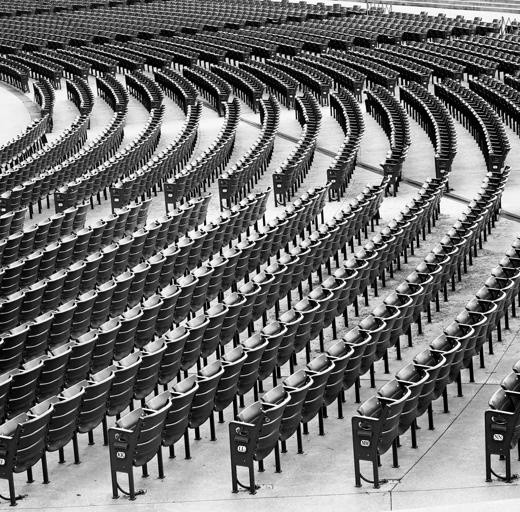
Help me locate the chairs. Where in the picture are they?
[0,0,520,511]
